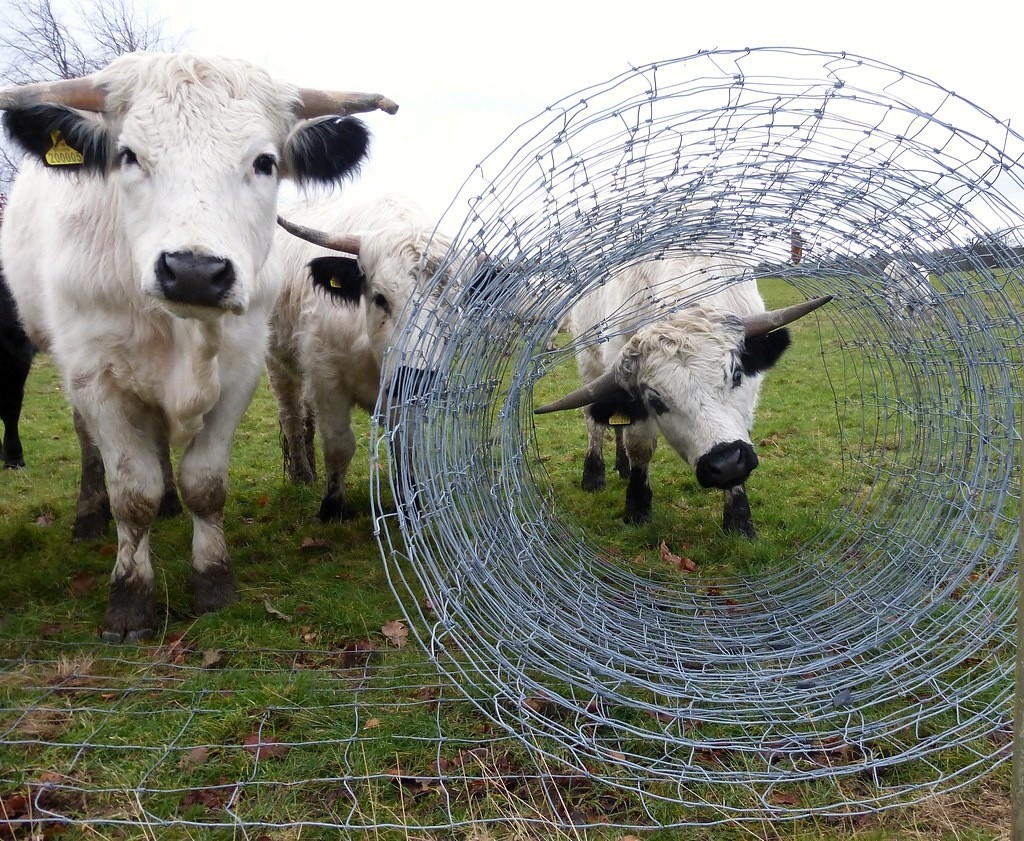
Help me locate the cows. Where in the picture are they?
[1,46,938,639]
